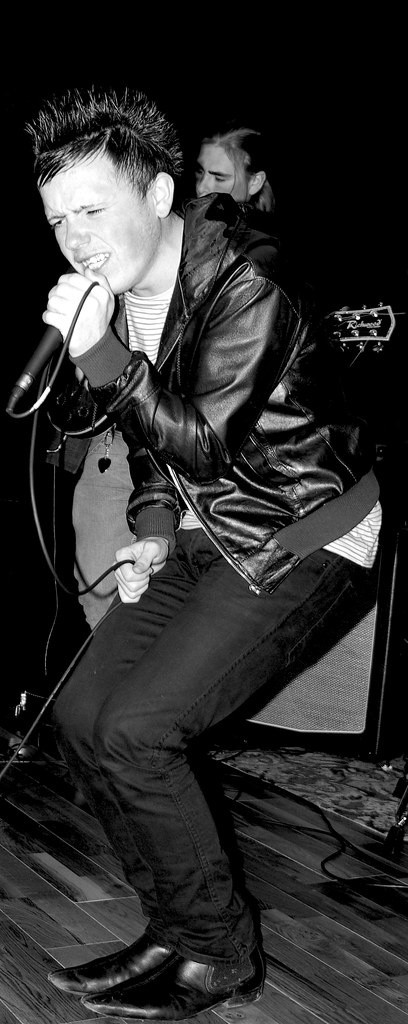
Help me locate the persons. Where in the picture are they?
[28,84,381,1020]
[70,124,275,635]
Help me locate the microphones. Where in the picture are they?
[6,271,98,409]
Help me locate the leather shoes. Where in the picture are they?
[47,928,173,996]
[80,938,265,1022]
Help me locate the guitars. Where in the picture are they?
[42,299,397,440]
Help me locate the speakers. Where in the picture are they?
[227,523,404,764]
